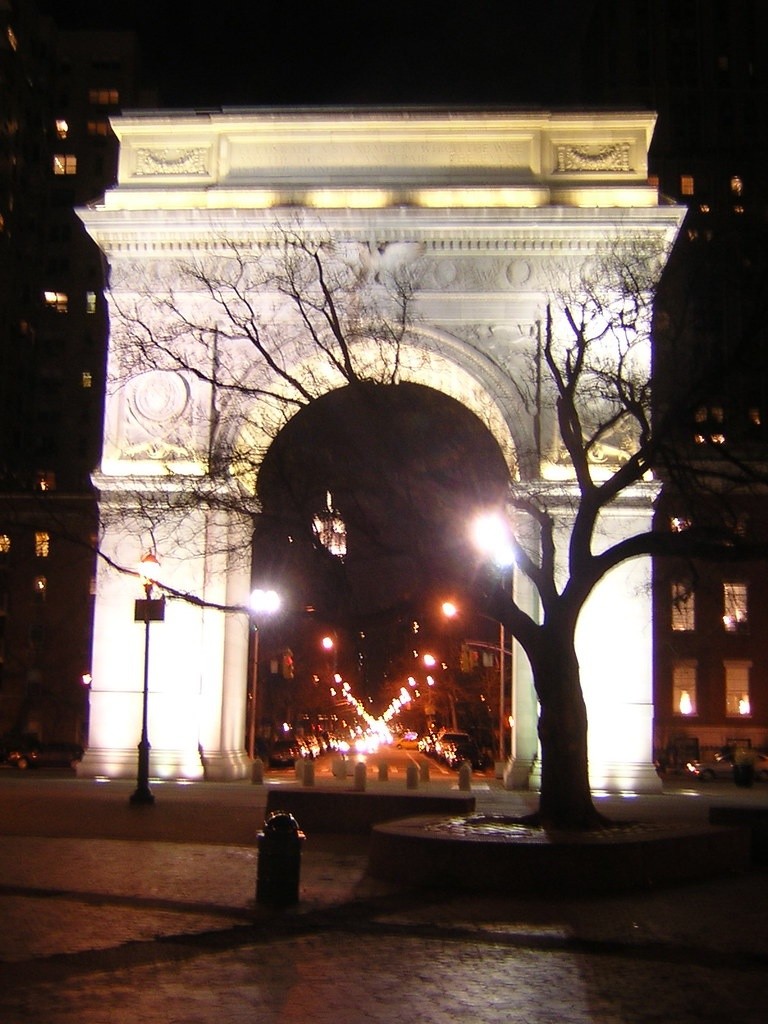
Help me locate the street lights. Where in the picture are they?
[130,549,166,804]
[322,625,338,729]
[441,600,506,763]
[249,588,281,762]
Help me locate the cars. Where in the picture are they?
[687,748,768,783]
[8,737,83,772]
[267,720,495,770]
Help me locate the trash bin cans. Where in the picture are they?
[254,812,302,908]
